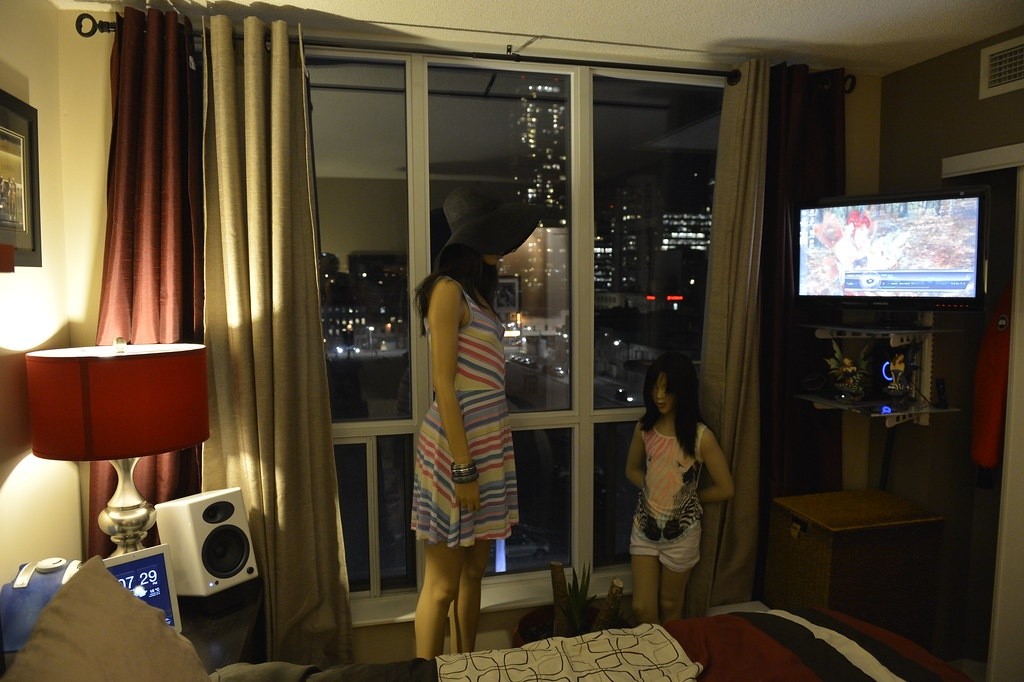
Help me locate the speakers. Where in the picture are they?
[153,487,259,596]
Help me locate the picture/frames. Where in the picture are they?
[0,89,42,267]
[491,276,521,314]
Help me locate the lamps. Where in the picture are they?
[26,339,212,560]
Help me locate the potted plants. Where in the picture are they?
[511,562,642,651]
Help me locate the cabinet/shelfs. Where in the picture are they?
[794,322,964,427]
[761,487,946,653]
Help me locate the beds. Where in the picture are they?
[0,555,982,682]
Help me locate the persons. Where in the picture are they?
[624,351,735,625]
[409,169,546,660]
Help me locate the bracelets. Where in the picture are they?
[450,459,480,484]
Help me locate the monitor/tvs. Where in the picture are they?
[792,186,987,310]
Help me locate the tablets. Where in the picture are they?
[104,544,182,634]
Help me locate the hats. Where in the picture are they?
[441,180,549,258]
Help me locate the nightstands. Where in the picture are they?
[175,575,268,675]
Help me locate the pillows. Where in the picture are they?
[2,555,213,681]
